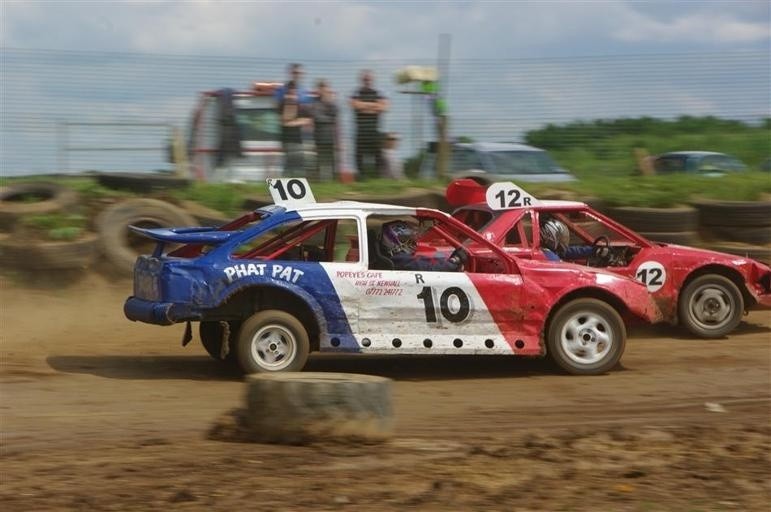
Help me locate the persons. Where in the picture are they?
[300,79,345,181]
[369,219,470,273]
[279,79,316,178]
[345,67,391,179]
[276,61,315,107]
[378,130,409,180]
[538,217,614,266]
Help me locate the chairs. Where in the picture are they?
[368,229,395,270]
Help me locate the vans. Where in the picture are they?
[159,79,346,187]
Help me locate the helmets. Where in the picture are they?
[538,217,570,256]
[376,219,420,257]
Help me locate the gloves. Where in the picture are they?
[447,247,470,264]
[594,247,615,261]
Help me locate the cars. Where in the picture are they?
[342,194,771,340]
[420,138,578,184]
[123,173,665,380]
[623,147,754,180]
